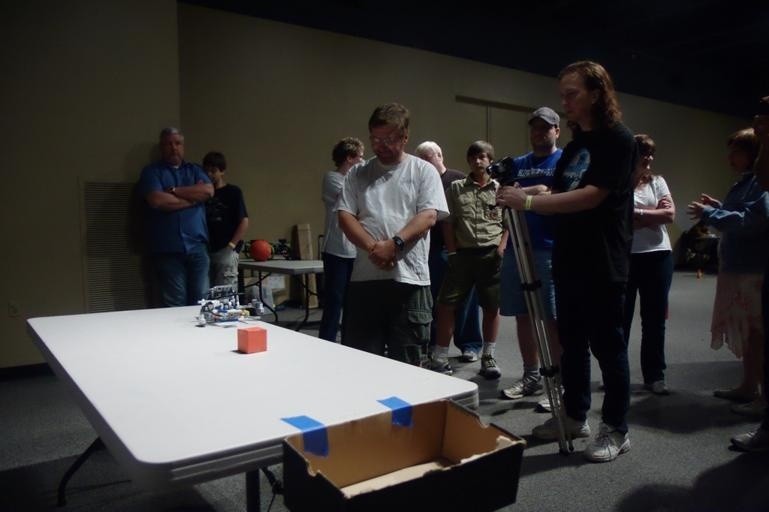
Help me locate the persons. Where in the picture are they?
[317,136,366,347]
[141,127,216,307]
[684,126,768,415]
[729,94,768,452]
[444,139,510,380]
[200,152,250,304]
[594,131,677,398]
[496,106,564,413]
[414,139,467,369]
[495,59,639,466]
[329,100,451,368]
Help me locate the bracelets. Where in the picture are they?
[524,194,533,212]
[227,241,236,250]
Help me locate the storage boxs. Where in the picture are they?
[281,396,528,511]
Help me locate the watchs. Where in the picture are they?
[168,186,177,195]
[392,237,404,253]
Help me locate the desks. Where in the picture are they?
[26,301,480,511]
[237,259,325,327]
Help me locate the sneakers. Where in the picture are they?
[501,370,544,399]
[583,421,633,463]
[729,426,769,453]
[731,399,768,416]
[644,378,669,395]
[532,413,592,442]
[421,354,454,376]
[462,349,479,362]
[480,355,501,379]
[713,381,758,402]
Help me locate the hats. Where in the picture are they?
[529,106,561,126]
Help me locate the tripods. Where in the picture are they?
[487,156,574,454]
